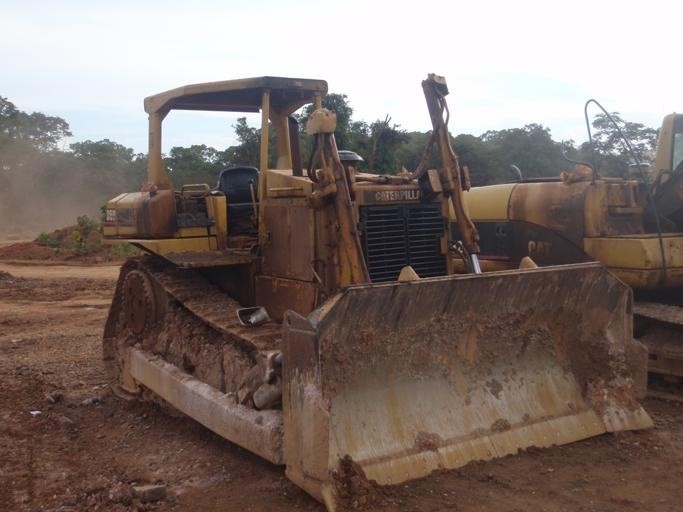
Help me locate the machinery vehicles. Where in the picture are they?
[98,73,655,511]
[447,94,681,406]
